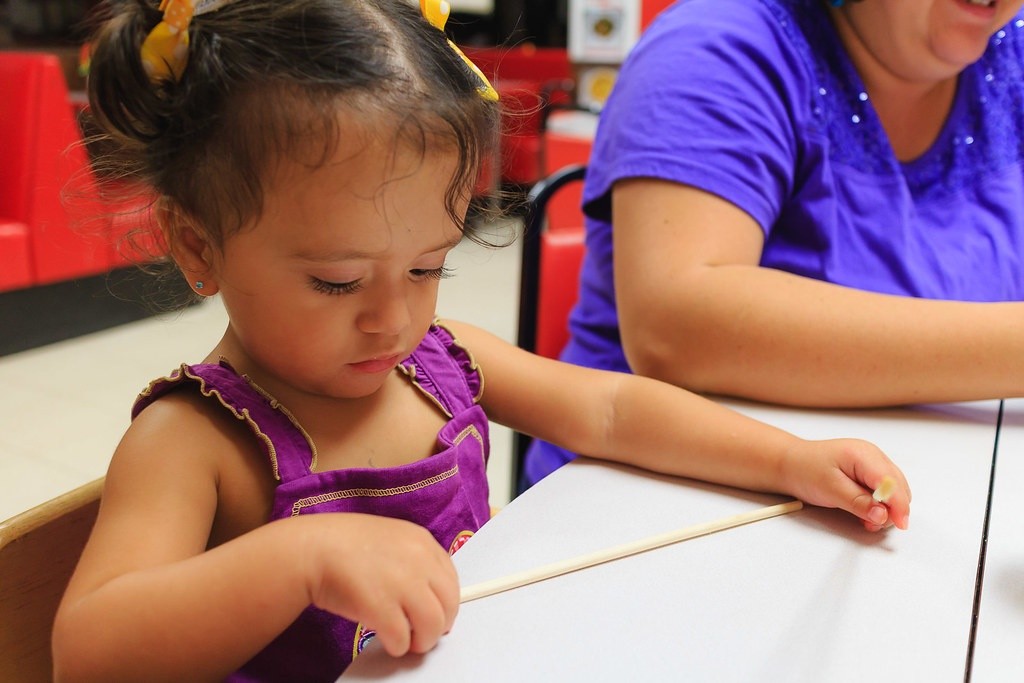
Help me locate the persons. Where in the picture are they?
[524,0,1024,492]
[55,0,910,683]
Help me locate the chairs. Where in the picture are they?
[1,478,106,683]
[506,161,590,497]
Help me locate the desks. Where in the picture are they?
[336,383,1024,683]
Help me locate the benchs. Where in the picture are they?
[0,1,578,292]
